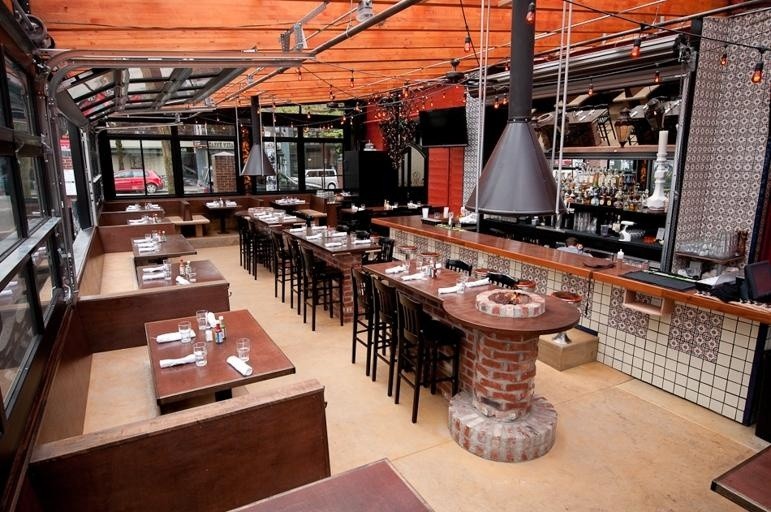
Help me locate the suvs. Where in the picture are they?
[289,168,339,190]
[113,168,165,195]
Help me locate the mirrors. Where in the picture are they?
[533,75,685,152]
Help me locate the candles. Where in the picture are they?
[657,128,670,153]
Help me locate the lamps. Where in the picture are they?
[292,0,374,50]
[456,2,510,112]
[294,62,362,102]
[522,1,771,94]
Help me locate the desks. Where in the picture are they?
[709,444,770,512]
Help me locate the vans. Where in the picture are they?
[197,166,310,192]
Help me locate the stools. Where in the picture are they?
[514,277,537,291]
[550,290,583,345]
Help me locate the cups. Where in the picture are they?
[178,321,192,343]
[163,258,172,281]
[421,206,466,228]
[457,278,466,294]
[190,272,198,282]
[193,340,208,367]
[527,213,622,238]
[312,223,359,243]
[351,199,422,214]
[196,310,209,329]
[144,229,168,253]
[236,338,251,361]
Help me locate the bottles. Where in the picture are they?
[664,197,670,212]
[610,248,625,271]
[403,250,440,279]
[558,164,649,212]
[203,316,229,342]
[180,259,192,279]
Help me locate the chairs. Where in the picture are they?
[533,82,683,147]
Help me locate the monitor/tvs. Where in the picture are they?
[744,260,771,299]
[418,106,468,148]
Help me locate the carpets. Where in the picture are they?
[230,457,436,512]
